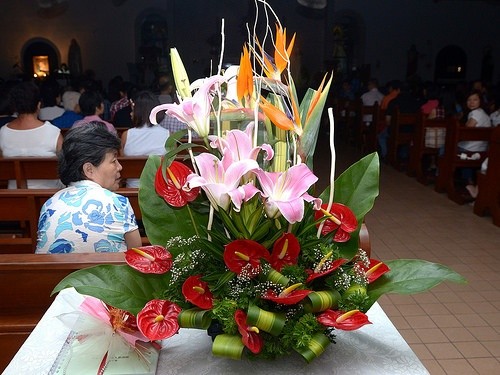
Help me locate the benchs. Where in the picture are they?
[328,95,499,228]
[0,127,216,375]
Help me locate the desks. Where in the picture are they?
[0,282,432,375]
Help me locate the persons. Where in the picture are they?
[118,89,177,237]
[35,123,142,254]
[0,60,177,128]
[335,75,500,208]
[0,88,63,234]
[67,91,118,137]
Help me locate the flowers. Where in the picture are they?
[48,0,470,365]
